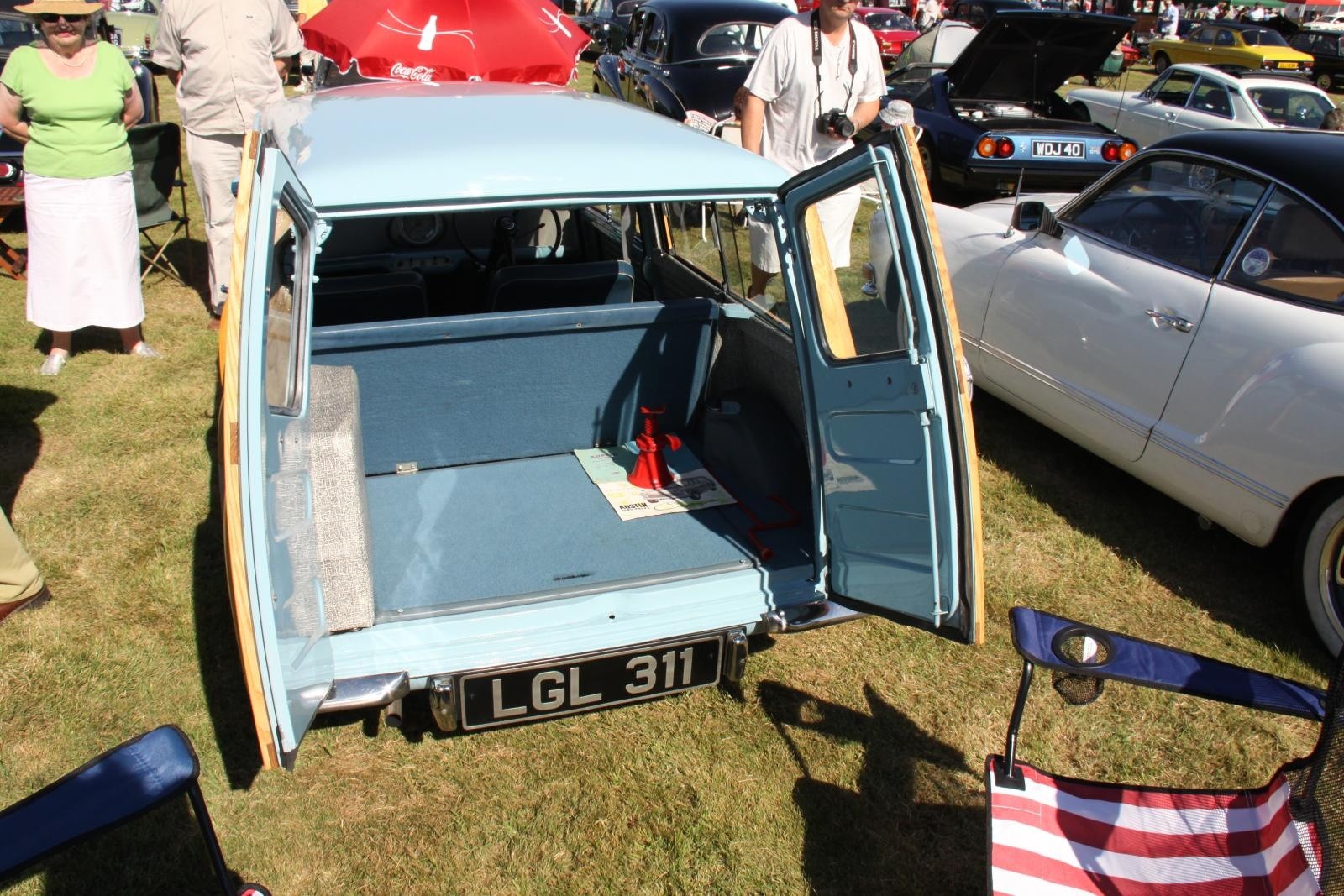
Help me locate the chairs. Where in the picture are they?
[1259,92,1287,118]
[1249,203,1344,303]
[980,607,1344,896]
[486,260,635,312]
[1206,90,1230,118]
[0,727,266,895]
[127,120,196,290]
[300,273,438,332]
[1095,52,1128,91]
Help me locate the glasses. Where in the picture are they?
[38,13,84,22]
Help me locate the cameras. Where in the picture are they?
[817,108,854,137]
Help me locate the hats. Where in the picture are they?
[14,0,104,15]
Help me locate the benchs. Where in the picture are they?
[310,299,724,478]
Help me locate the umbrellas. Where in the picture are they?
[298,0,595,87]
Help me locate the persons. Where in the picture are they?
[574,0,614,19]
[912,0,938,35]
[1318,108,1344,131]
[1021,0,1322,68]
[617,53,625,82]
[641,81,654,110]
[740,0,888,303]
[1,0,163,375]
[937,0,945,20]
[293,0,329,92]
[1187,173,1250,275]
[151,0,305,332]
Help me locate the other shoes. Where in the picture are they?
[40,351,71,376]
[748,285,766,310]
[0,582,52,625]
[123,343,163,360]
[208,316,221,331]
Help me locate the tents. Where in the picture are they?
[1173,0,1288,8]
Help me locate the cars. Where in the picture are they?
[855,8,1344,659]
[0,14,158,172]
[570,0,799,140]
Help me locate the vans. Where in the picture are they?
[214,77,986,770]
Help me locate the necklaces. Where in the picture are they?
[48,34,88,67]
